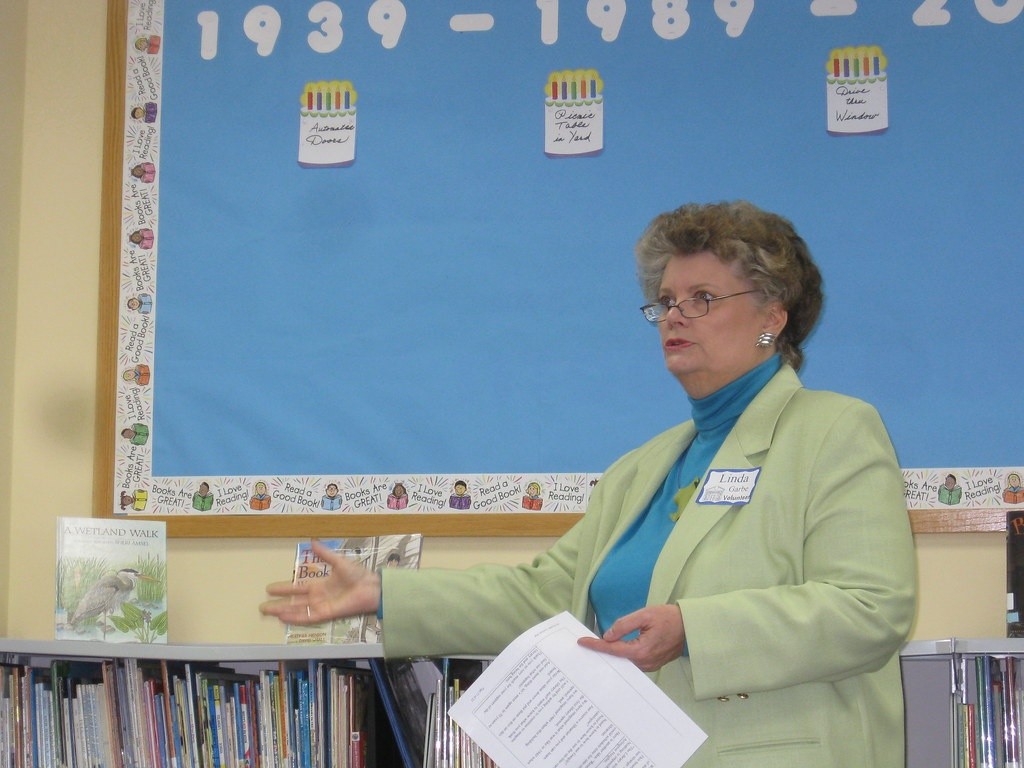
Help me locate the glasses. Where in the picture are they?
[639,288,763,323]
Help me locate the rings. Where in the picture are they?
[306,606,310,615]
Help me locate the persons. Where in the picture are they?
[260,201,920,768]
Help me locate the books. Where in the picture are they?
[0,659,498,768]
[949,654,1024,768]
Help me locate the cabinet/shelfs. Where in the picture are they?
[0,638,1024,768]
